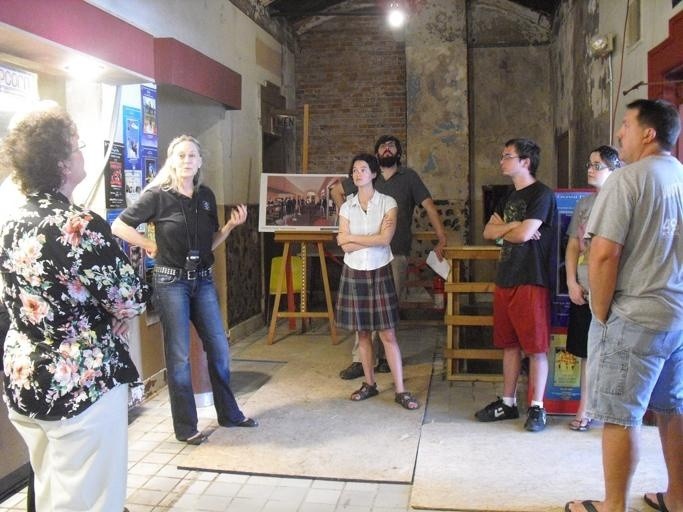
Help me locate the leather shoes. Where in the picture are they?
[237,417,257,427]
[185,433,206,445]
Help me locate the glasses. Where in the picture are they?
[586,162,606,170]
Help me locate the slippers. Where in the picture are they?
[564,500,598,512]
[643,492,667,512]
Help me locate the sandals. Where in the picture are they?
[567,417,590,431]
[394,392,419,410]
[350,383,378,400]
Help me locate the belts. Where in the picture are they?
[153,265,213,281]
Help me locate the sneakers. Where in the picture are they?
[474,400,518,421]
[523,405,546,432]
[339,361,364,379]
[377,359,390,372]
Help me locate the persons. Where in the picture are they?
[337,151,420,410]
[475,138,558,432]
[566,98,682,512]
[1,101,148,512]
[330,135,448,380]
[110,134,260,445]
[567,145,623,431]
[266,195,333,217]
